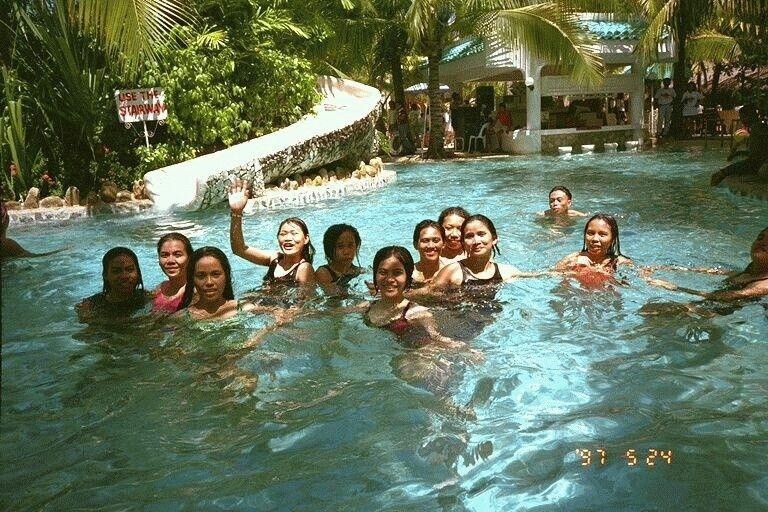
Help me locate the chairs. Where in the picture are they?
[468,121,491,153]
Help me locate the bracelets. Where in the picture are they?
[230,212,244,217]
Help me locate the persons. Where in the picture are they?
[182,245,236,314]
[710,103,768,187]
[555,212,636,289]
[384,90,515,156]
[438,206,469,261]
[226,179,316,282]
[533,186,588,234]
[1,204,71,277]
[435,215,515,283]
[637,224,768,323]
[83,246,148,308]
[679,81,707,140]
[314,224,362,280]
[368,246,424,316]
[155,232,196,296]
[651,78,677,141]
[412,221,453,277]
[727,137,751,161]
